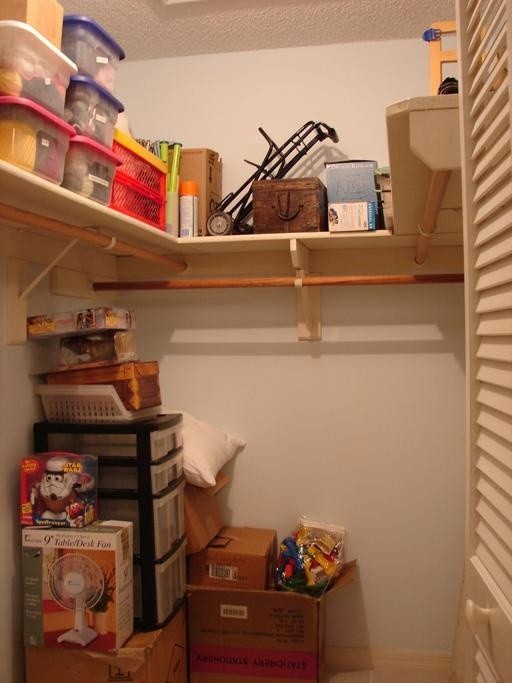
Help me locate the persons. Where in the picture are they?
[28,455,95,525]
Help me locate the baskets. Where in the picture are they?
[33,381,160,425]
[109,175,167,233]
[108,139,168,203]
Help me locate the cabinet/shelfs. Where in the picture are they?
[32,411,189,633]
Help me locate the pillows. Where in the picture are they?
[177,413,248,489]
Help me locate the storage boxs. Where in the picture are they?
[1,0,123,205]
[19,520,188,682]
[169,143,378,233]
[181,470,357,682]
